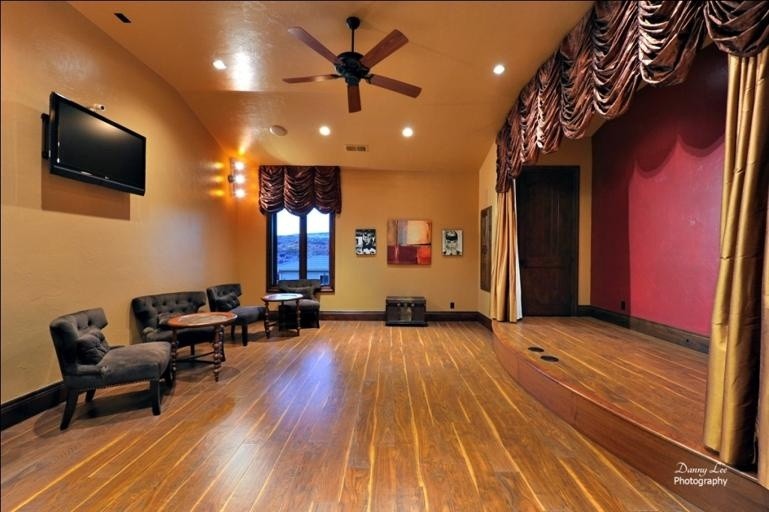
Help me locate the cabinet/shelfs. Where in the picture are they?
[385,296,427,326]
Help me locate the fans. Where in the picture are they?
[282,16,421,113]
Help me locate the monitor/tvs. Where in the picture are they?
[49,91,146,197]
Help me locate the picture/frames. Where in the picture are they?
[354,228,376,255]
[441,229,462,256]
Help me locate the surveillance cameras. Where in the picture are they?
[88,102,105,113]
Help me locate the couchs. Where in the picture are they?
[50,307,171,431]
[132,292,225,361]
[207,283,267,345]
[277,280,321,329]
